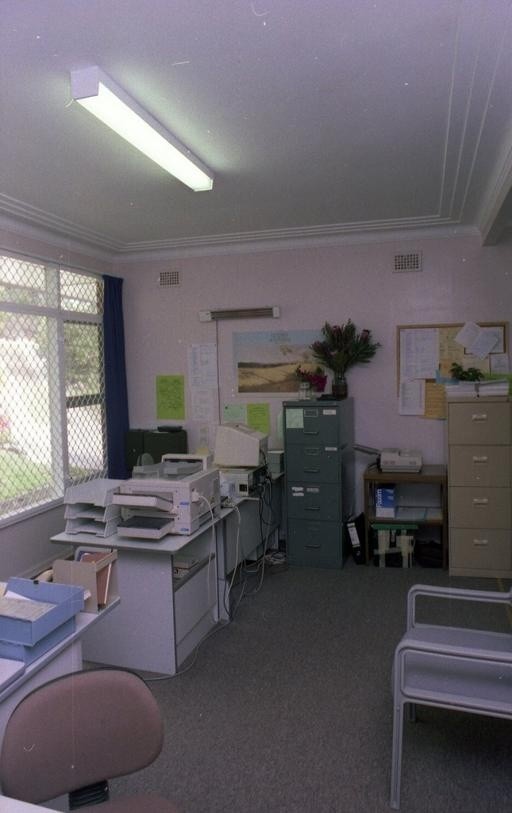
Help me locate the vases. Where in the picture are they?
[298,381,312,400]
[331,372,347,399]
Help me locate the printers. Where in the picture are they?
[352,443,424,473]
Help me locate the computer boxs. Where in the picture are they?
[215,466,272,497]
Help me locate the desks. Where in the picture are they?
[0,578,121,751]
[50,472,285,676]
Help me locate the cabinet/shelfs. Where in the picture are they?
[364,465,448,571]
[283,398,354,570]
[446,397,512,579]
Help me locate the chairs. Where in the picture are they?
[390,584,512,810]
[0,666,182,813]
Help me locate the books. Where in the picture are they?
[443,377,508,397]
[112,491,173,511]
[117,516,176,539]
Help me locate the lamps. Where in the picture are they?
[70,66,214,193]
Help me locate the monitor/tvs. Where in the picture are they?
[160,453,213,469]
[214,423,269,468]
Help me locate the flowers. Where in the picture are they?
[295,366,328,392]
[308,317,384,384]
